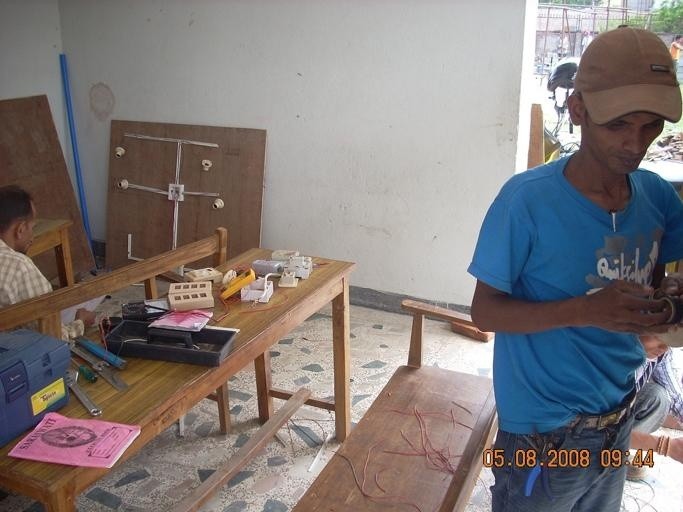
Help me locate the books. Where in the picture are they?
[147,309,213,333]
[10,411,142,471]
[59,294,106,324]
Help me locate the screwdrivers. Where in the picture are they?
[70,357,98,383]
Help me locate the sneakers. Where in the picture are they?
[627,454,647,482]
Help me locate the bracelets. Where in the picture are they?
[656,435,671,458]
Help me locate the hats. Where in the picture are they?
[574,24,683,124]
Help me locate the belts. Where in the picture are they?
[566,390,641,430]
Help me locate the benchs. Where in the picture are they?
[293,299,499,512]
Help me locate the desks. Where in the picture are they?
[1,245,356,510]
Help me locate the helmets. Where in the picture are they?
[548,63,578,92]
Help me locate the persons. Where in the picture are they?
[464,22,682,512]
[581,31,592,55]
[0,183,97,347]
[557,33,569,62]
[668,35,683,74]
[624,333,683,481]
